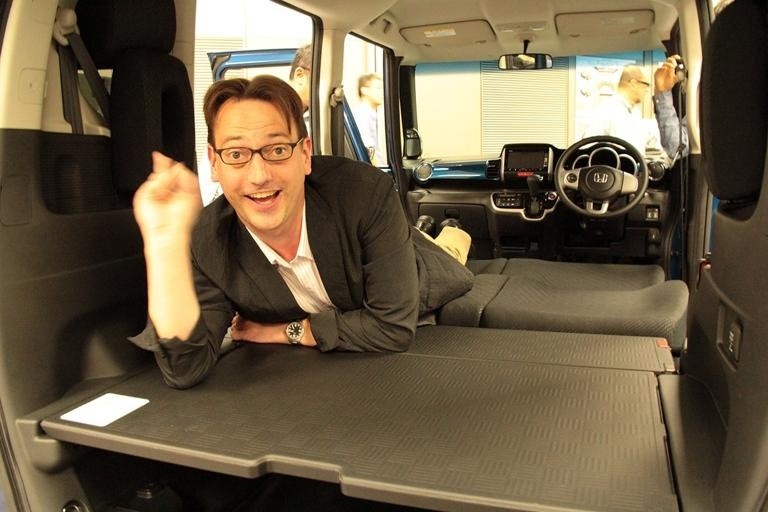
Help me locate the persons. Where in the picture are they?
[647,51,692,182]
[348,74,389,172]
[126,70,475,393]
[578,65,660,161]
[211,42,313,200]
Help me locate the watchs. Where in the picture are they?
[283,314,306,348]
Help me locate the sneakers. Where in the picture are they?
[440,218,477,259]
[415,215,436,238]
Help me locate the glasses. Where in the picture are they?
[212,133,307,165]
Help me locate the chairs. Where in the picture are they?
[438,256,692,350]
